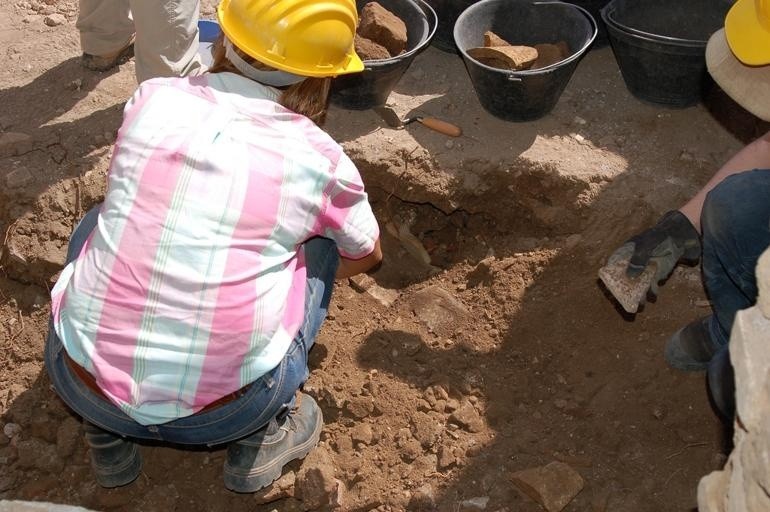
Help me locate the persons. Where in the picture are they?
[42,0,384,494]
[606,0,770,419]
[73,0,209,86]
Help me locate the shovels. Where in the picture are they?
[373,106,462,136]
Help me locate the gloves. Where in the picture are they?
[605,210,702,313]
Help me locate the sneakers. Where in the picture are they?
[81,34,136,71]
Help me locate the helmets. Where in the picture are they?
[216,0,365,78]
[725,0,769,66]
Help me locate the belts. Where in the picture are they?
[62,344,250,417]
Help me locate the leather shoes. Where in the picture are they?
[222,391,323,494]
[83,429,141,489]
[667,314,719,372]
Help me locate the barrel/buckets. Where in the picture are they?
[331,0,438,111]
[601,3,731,110]
[452,0,598,123]
